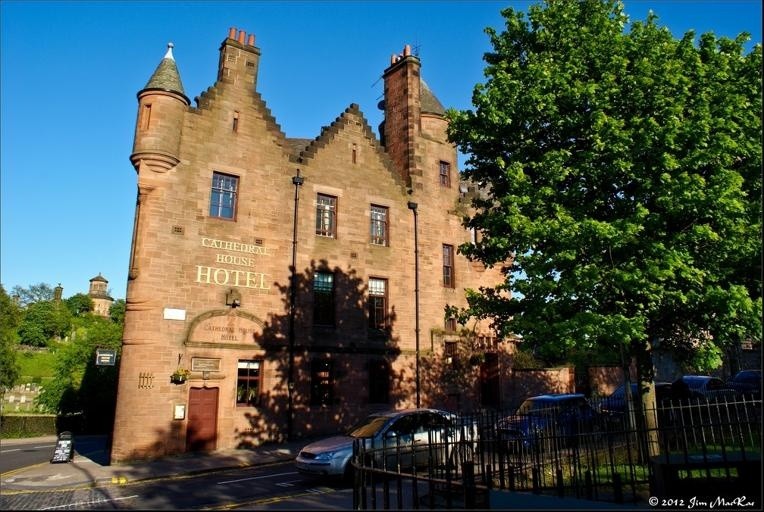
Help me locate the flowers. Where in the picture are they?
[174,368,186,376]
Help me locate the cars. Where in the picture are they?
[294,408,480,480]
[494,368,762,455]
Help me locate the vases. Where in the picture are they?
[174,374,185,383]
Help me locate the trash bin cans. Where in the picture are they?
[647,455,764,512]
